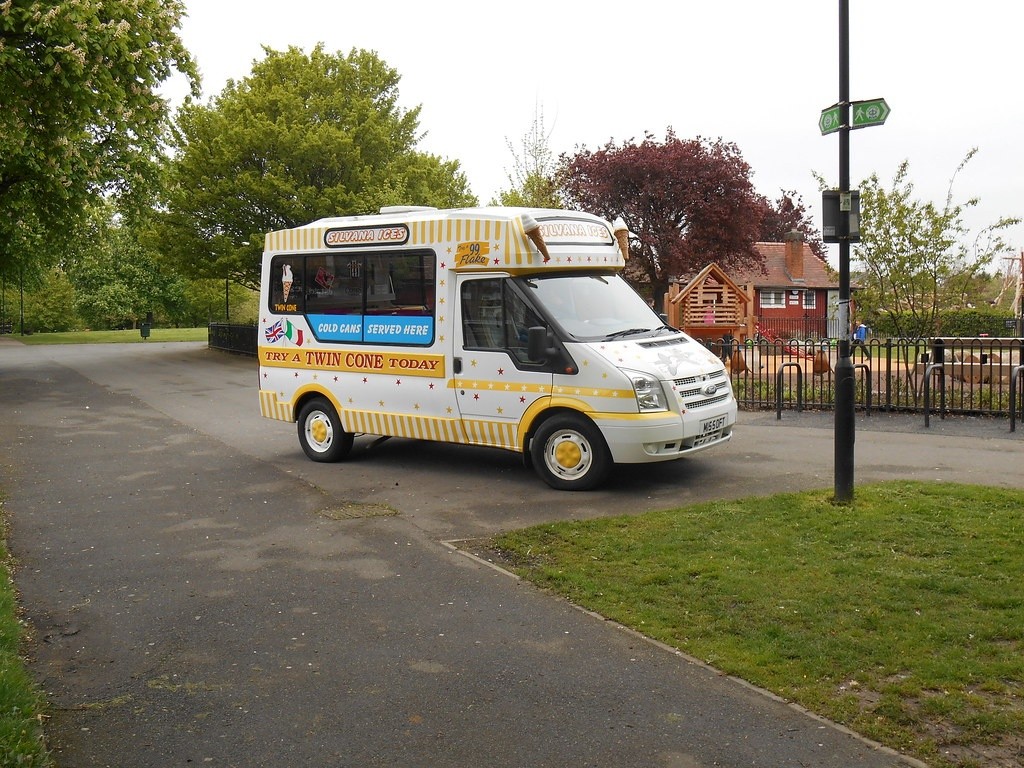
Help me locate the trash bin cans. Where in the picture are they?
[140,323,151,337]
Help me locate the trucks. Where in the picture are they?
[258,204,737,497]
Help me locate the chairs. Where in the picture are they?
[473,281,529,362]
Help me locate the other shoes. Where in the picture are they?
[865,357,871,361]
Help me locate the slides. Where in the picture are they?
[753,320,816,361]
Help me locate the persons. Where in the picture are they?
[701,306,715,324]
[849,320,870,361]
[721,334,732,362]
[647,298,654,309]
